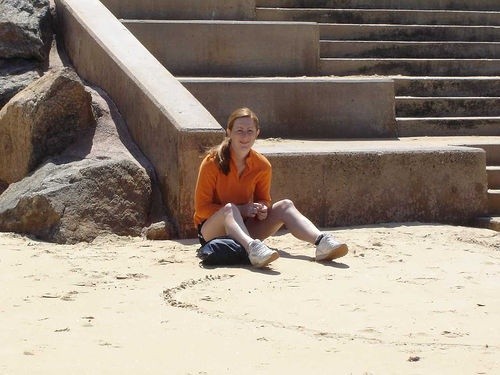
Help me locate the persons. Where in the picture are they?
[193,108,349,268]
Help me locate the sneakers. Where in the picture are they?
[248,239,280,268]
[316,235,348,260]
